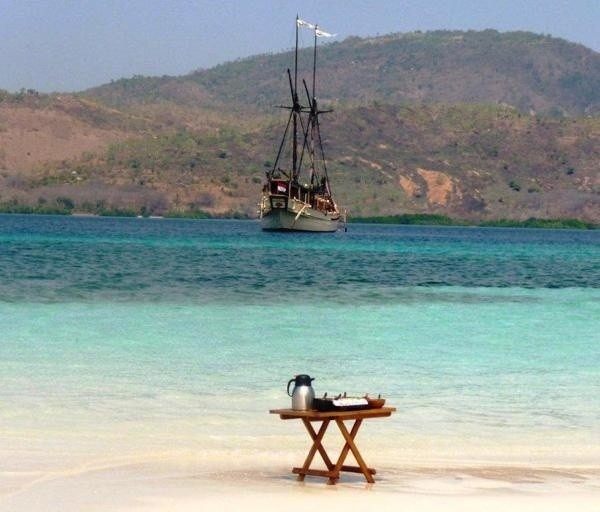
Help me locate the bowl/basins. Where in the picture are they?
[369,399,385,408]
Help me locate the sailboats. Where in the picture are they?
[257,12,348,234]
